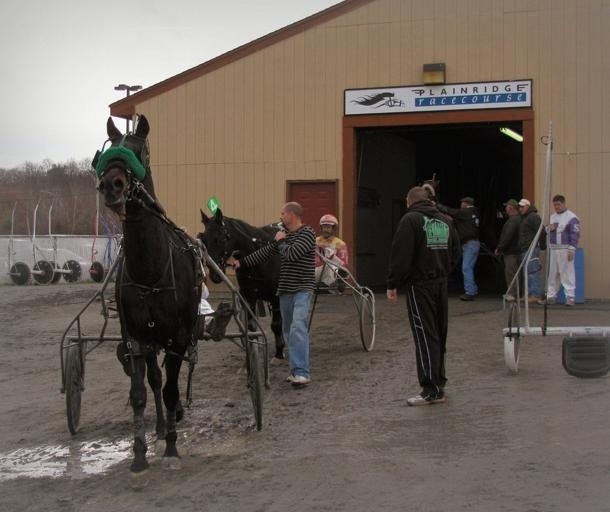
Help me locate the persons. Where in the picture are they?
[517,198,547,303]
[431,197,480,301]
[537,195,580,306]
[494,199,522,301]
[314,214,349,294]
[197,279,235,342]
[386,186,462,406]
[231,200,316,386]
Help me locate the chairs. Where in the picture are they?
[561,336,609,379]
[314,265,348,295]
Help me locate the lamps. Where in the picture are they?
[422,62,446,85]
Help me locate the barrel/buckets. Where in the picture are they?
[547,247,586,305]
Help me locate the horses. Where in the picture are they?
[416,176,440,203]
[200,207,286,366]
[96,113,204,478]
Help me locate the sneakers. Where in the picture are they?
[285,373,313,387]
[565,297,576,306]
[502,295,545,304]
[406,389,448,406]
[337,267,349,291]
[459,293,482,301]
[536,297,558,306]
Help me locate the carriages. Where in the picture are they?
[55,117,270,476]
[192,198,381,362]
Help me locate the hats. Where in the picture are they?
[460,197,474,203]
[503,199,519,208]
[318,213,339,228]
[518,198,531,207]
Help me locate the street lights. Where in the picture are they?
[109,79,143,137]
